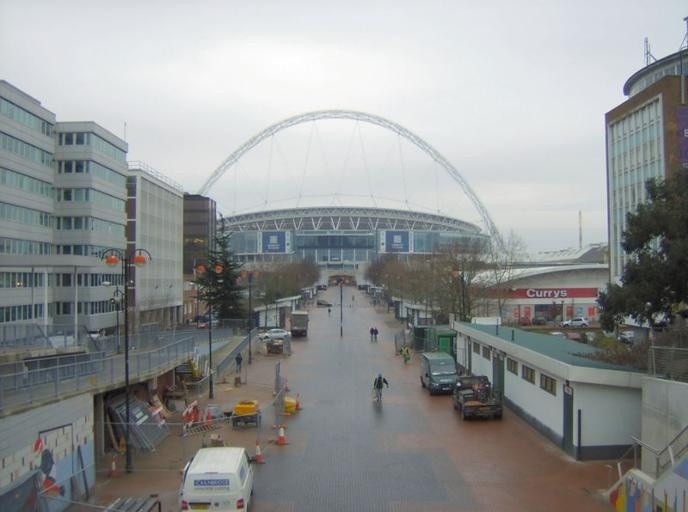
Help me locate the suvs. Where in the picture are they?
[559,317,589,329]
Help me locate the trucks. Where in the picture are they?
[451,376,503,421]
[291,311,308,337]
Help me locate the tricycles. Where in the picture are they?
[232,399,261,428]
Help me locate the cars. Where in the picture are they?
[520,316,545,325]
[621,331,633,342]
[258,327,291,340]
[189,316,219,329]
[317,299,333,306]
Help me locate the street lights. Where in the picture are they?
[452,271,465,321]
[337,276,345,336]
[195,262,224,397]
[240,270,262,363]
[99,247,151,471]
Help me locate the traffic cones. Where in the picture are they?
[110,456,117,477]
[255,440,264,464]
[277,427,287,445]
[296,393,302,410]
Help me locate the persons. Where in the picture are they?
[235,353,242,372]
[374,373,389,403]
[370,326,378,342]
[400,343,410,364]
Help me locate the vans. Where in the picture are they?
[178,446,253,512]
[420,351,459,395]
